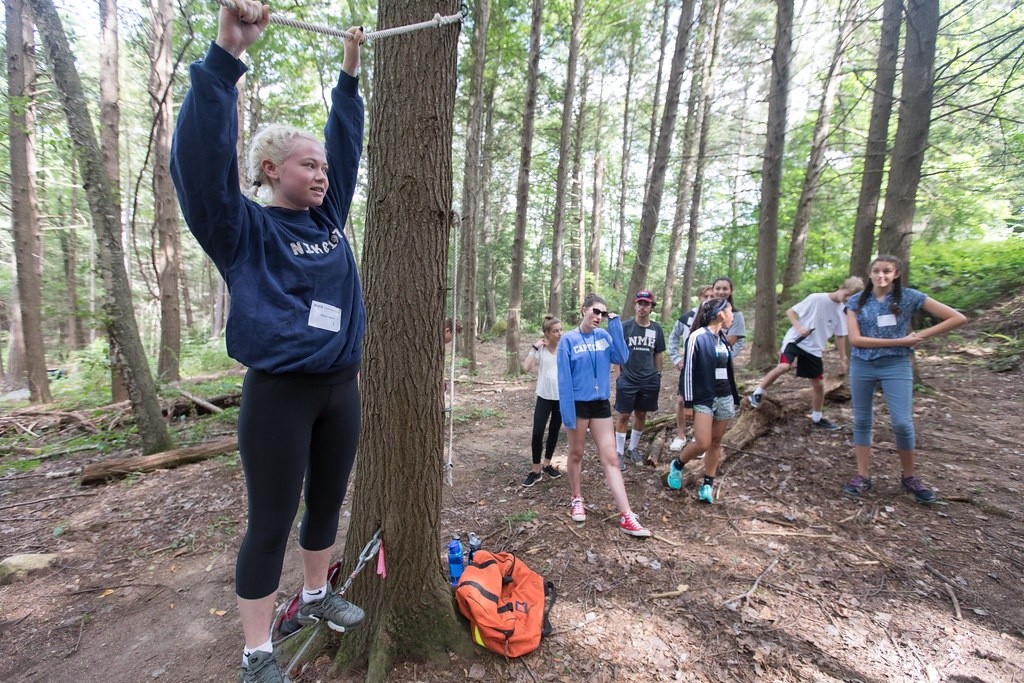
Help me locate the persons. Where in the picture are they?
[667,298,740,504]
[668,285,713,458]
[751,276,864,430]
[712,277,746,357]
[614,291,666,471]
[557,293,650,537]
[843,256,966,504]
[446,317,463,343]
[522,315,562,487]
[170,0,366,683]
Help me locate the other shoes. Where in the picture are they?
[670,435,686,450]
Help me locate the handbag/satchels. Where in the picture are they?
[454,547,557,657]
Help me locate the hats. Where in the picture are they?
[635,289,655,302]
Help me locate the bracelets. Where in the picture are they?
[532,345,538,351]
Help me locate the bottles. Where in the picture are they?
[468,532,482,565]
[448,536,465,586]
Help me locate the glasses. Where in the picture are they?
[636,292,651,298]
[587,307,609,318]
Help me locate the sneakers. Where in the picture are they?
[667,460,683,489]
[571,497,586,521]
[697,484,713,503]
[297,581,366,628]
[521,471,542,486]
[844,475,870,494]
[542,465,561,478]
[748,392,763,407]
[626,446,641,464]
[811,418,842,430]
[901,474,937,502]
[617,452,626,471]
[239,649,291,683]
[618,511,651,536]
